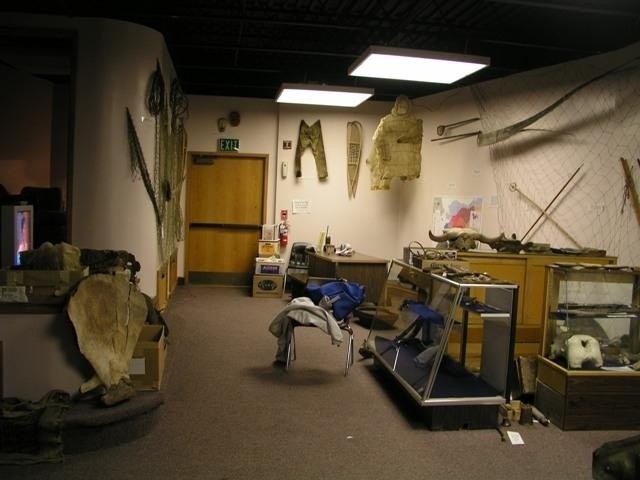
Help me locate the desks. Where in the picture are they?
[308,250,389,305]
[420,253,618,343]
[0,301,94,403]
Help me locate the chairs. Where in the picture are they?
[283,242,310,294]
[285,276,354,377]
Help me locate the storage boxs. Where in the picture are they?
[252,275,283,298]
[6,265,89,305]
[129,324,167,392]
[258,240,280,258]
[255,258,286,275]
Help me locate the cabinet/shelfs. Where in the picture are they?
[365,259,519,431]
[533,262,640,431]
[184,151,269,289]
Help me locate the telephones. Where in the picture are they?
[326,227,331,245]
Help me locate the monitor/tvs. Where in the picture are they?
[0,205,35,268]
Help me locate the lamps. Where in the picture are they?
[274,82,375,109]
[348,44,493,86]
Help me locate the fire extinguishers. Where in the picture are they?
[279,220,291,245]
